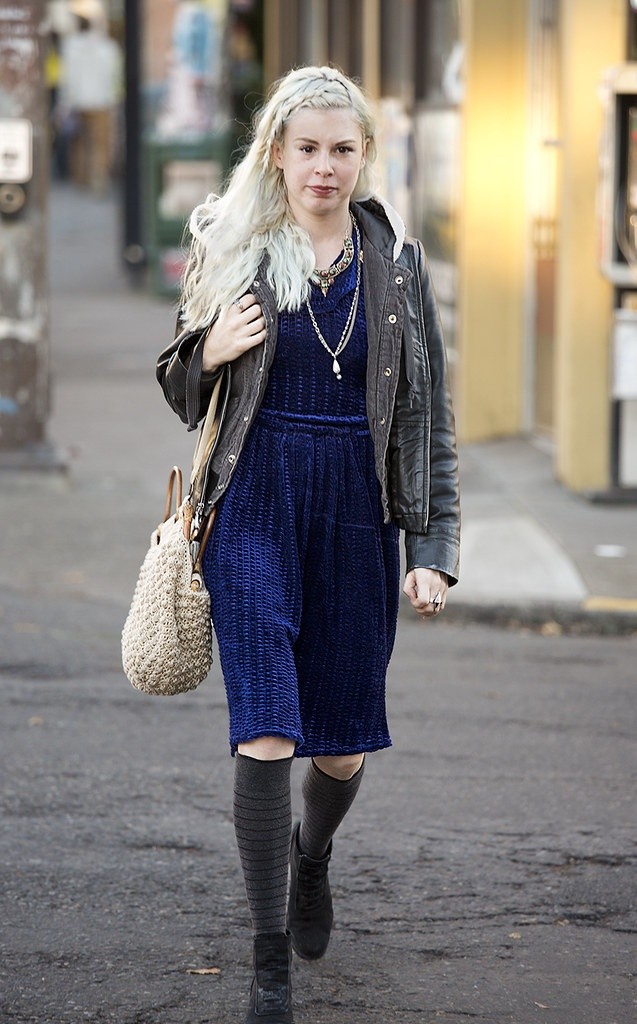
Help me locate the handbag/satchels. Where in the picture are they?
[122,465,216,698]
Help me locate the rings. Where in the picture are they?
[429,592,442,611]
[235,299,245,311]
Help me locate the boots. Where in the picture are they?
[248,929,295,1024]
[288,822,334,960]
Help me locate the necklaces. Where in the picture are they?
[306,209,364,380]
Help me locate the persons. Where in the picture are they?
[156,66,461,1024]
[44,16,123,192]
